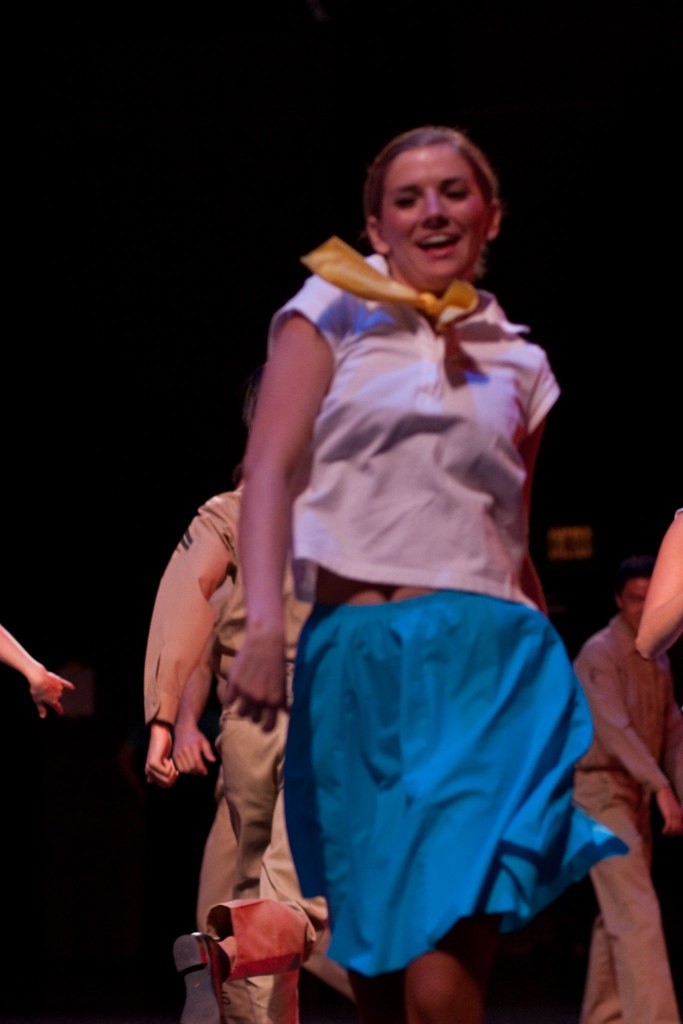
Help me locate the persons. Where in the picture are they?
[636,507,683,660]
[144,462,358,1024]
[222,128,631,1024]
[0,623,75,719]
[116,724,148,803]
[573,555,683,1024]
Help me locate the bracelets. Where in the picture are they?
[151,719,174,733]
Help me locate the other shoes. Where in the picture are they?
[174,932,226,1024]
